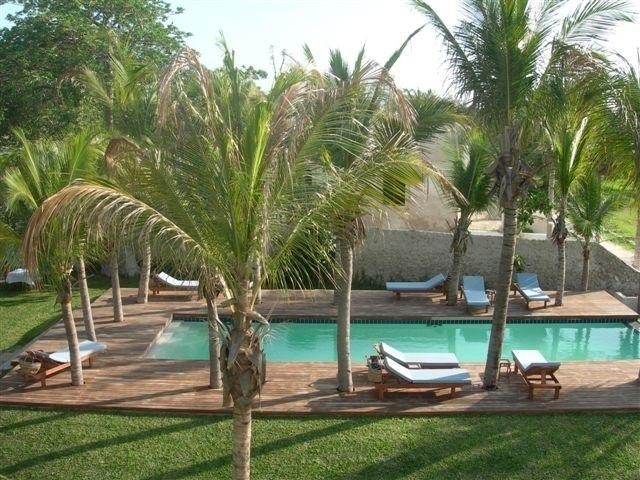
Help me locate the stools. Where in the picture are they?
[486,289,497,304]
[498,358,511,381]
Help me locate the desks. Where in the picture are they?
[5,268,36,290]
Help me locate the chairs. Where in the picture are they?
[367,355,470,401]
[366,340,463,370]
[149,271,201,295]
[12,339,108,390]
[460,275,491,313]
[511,349,562,400]
[385,272,445,300]
[513,272,551,310]
